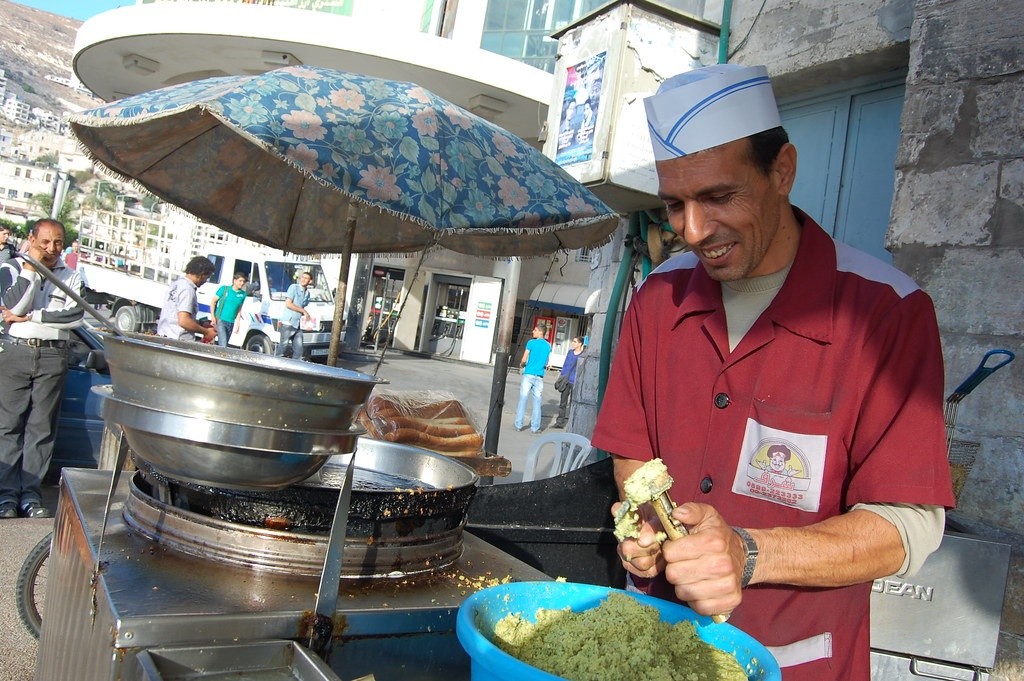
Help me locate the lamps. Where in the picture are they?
[468,94,507,119]
[543,271,549,281]
[122,55,159,76]
[261,50,303,71]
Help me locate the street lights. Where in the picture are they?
[90,180,170,279]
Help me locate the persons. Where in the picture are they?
[549,336,584,428]
[157,256,218,342]
[559,94,596,133]
[590,64,955,681]
[513,323,552,434]
[0,218,86,517]
[276,272,313,360]
[210,272,249,347]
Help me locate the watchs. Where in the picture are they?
[729,525,759,588]
[26,309,34,322]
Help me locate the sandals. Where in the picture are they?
[0,502,18,517]
[23,502,51,518]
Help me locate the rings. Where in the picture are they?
[4,315,7,318]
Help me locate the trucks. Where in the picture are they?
[76,246,347,363]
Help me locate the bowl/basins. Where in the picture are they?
[90,331,391,433]
[90,385,364,491]
[456,581,783,681]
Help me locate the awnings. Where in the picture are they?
[527,281,602,315]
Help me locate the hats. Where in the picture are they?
[643,63,782,161]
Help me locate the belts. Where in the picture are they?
[0,334,66,348]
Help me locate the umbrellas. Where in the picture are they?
[65,68,619,364]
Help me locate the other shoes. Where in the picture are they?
[531,429,538,433]
[549,423,563,429]
[514,428,522,432]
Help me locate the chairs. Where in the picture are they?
[522,433,593,482]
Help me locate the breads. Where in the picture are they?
[358,394,484,457]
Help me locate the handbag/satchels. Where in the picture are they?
[555,375,568,392]
